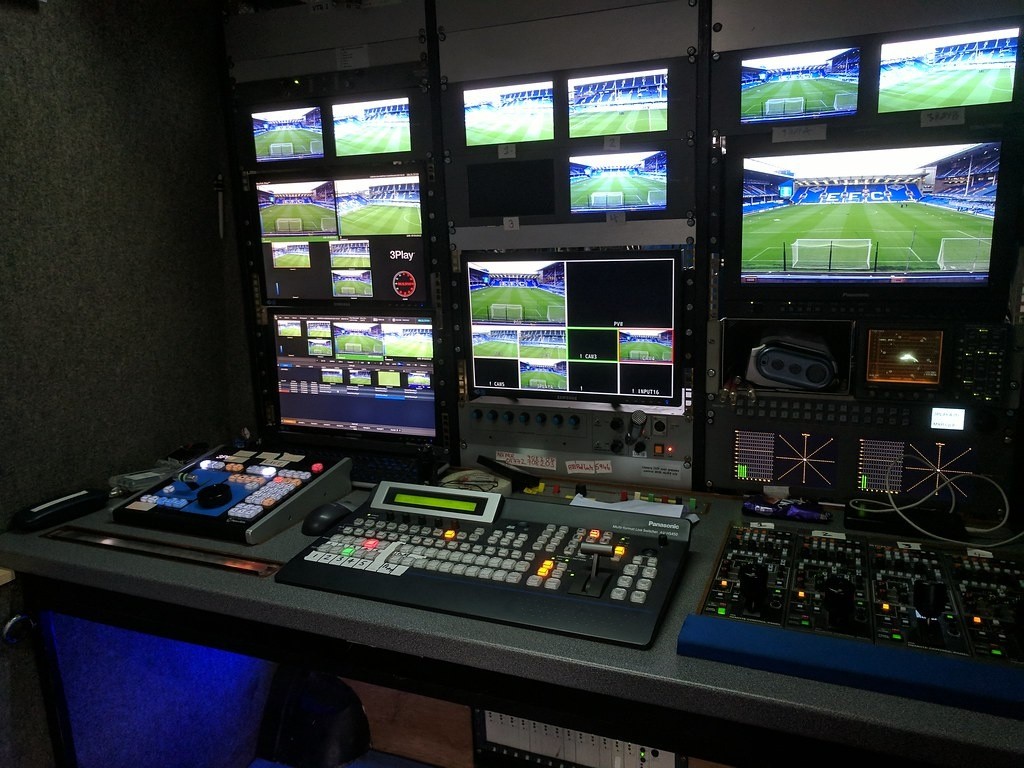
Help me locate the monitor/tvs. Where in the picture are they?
[248,14,1022,441]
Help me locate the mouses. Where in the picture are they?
[302,501,352,537]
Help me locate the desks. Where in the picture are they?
[1,444,1023,768]
